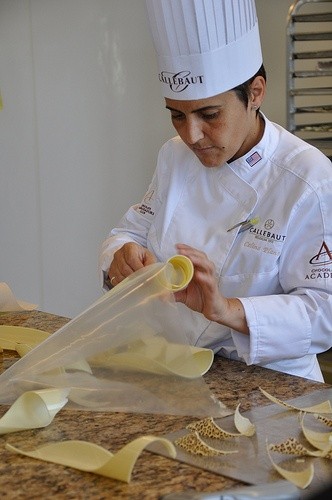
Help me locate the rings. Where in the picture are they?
[111,277,115,284]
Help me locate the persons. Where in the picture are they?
[96,0,332,383]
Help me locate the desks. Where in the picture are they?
[0,310,332,499]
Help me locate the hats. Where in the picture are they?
[144,0,262,101]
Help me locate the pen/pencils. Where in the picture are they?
[226,216,259,234]
